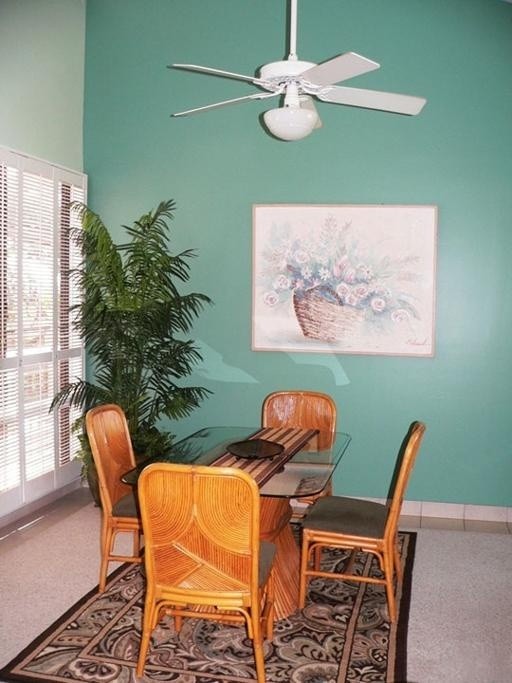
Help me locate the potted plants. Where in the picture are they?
[46,195,217,509]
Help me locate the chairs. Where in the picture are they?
[297,419,427,625]
[135,460,277,683]
[261,389,337,528]
[84,402,145,595]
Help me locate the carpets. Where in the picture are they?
[0,522,417,682]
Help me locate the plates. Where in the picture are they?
[225,440,284,462]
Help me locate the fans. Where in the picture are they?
[168,0,428,142]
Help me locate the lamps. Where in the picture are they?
[262,80,321,142]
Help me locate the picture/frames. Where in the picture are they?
[249,201,440,360]
